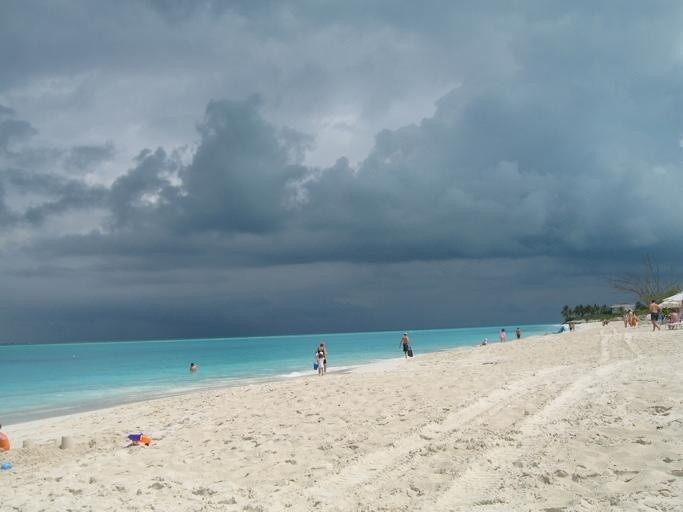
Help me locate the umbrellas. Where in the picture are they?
[661,291,683,308]
[658,301,680,315]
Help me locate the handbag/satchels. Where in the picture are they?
[408,346,413,357]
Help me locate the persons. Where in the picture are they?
[314,342,327,374]
[621,307,638,328]
[398,331,409,358]
[480,337,488,346]
[188,362,197,373]
[657,299,683,330]
[646,299,662,331]
[514,327,521,340]
[0,423,11,452]
[498,328,506,343]
[316,353,324,376]
[568,322,575,331]
